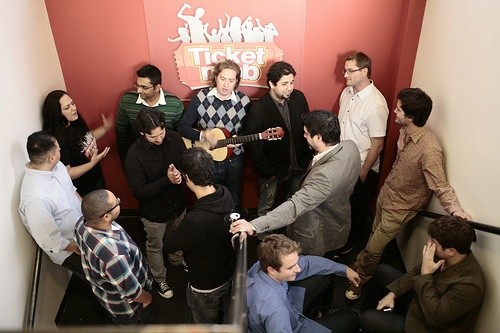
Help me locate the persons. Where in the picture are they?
[125,107,189,299]
[337,51,389,257]
[115,64,185,164]
[18,130,87,282]
[42,90,114,197]
[246,61,315,218]
[336,87,472,300]
[162,148,239,324]
[73,189,155,325]
[246,233,361,333]
[177,59,252,210]
[230,108,360,259]
[363,215,486,333]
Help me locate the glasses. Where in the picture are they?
[99,197,121,218]
[134,81,158,90]
[342,66,368,74]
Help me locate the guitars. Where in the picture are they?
[182,127,284,162]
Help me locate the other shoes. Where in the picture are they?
[340,238,358,255]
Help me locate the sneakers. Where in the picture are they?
[179,258,189,273]
[155,280,173,298]
[346,282,363,300]
